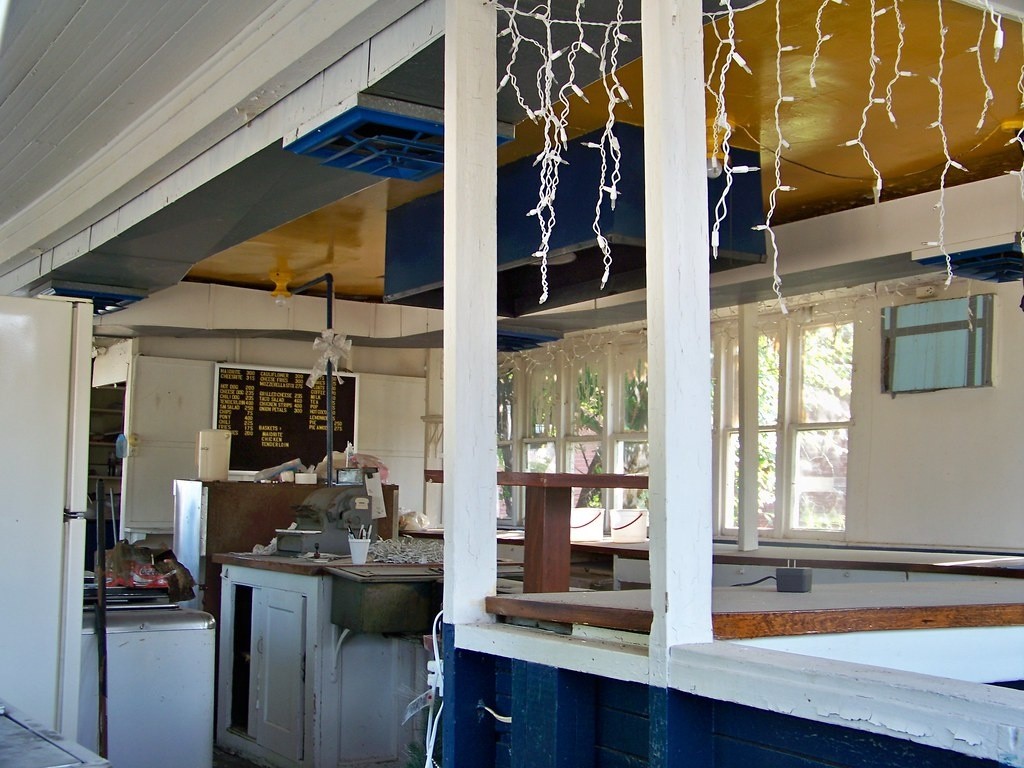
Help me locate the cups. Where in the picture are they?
[348,538,372,566]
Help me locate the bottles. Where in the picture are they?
[107,451,117,476]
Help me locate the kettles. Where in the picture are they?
[193,427,233,482]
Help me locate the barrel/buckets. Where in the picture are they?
[608,508,649,543]
[570,507,606,543]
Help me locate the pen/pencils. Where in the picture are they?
[347,524,373,540]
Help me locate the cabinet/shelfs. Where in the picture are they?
[155,465,1023,768]
